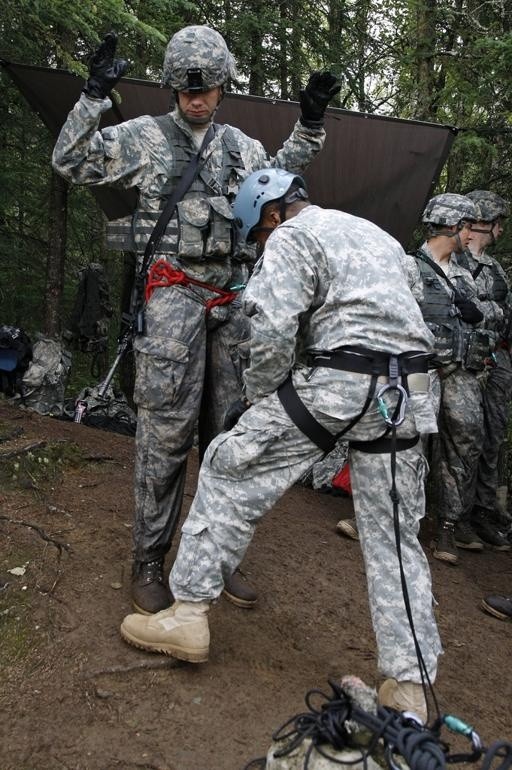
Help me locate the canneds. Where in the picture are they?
[74,400,87,423]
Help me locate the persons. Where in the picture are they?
[118,168,445,727]
[455,189,511,553]
[49,23,342,615]
[335,192,485,567]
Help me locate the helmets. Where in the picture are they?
[465,189,510,247]
[231,167,310,246]
[421,192,482,254]
[159,25,236,126]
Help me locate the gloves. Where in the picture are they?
[80,32,128,100]
[299,68,342,130]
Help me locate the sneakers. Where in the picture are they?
[375,678,429,727]
[119,559,211,664]
[453,514,485,551]
[220,567,258,610]
[430,515,459,563]
[336,516,360,540]
[469,503,512,552]
